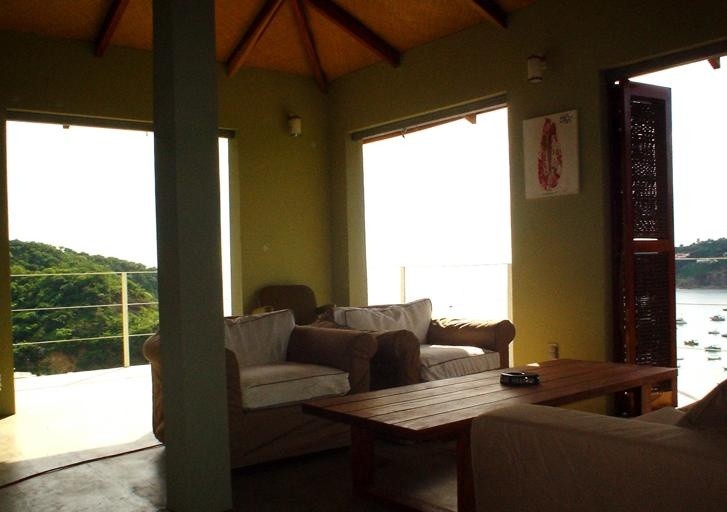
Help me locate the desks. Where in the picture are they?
[298,354,678,509]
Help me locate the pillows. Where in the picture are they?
[222,310,295,363]
[340,299,433,345]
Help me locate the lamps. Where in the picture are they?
[287,113,302,139]
[523,56,559,90]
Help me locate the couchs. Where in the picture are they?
[466,376,726,510]
[139,308,377,470]
[257,283,338,322]
[313,299,514,390]
